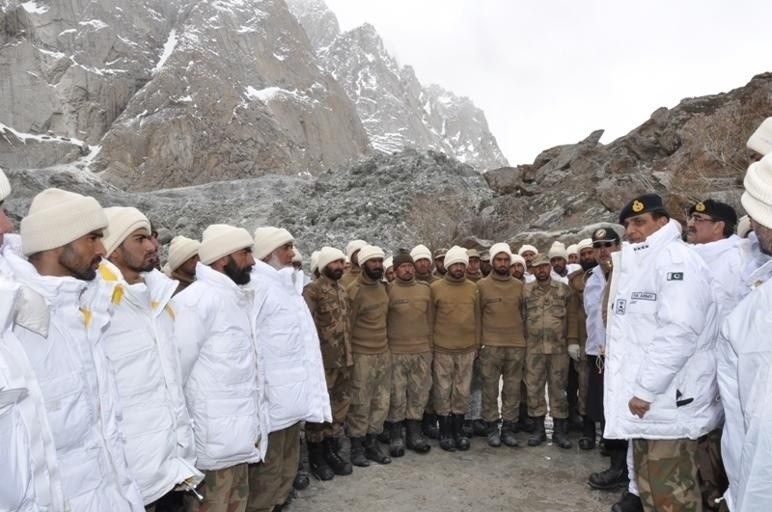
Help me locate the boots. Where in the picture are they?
[487,421,501,446]
[349,436,371,468]
[439,414,457,451]
[406,417,432,454]
[578,418,597,451]
[422,411,443,441]
[551,415,572,450]
[308,441,335,481]
[501,421,520,448]
[388,421,406,457]
[363,432,392,465]
[611,490,646,512]
[587,435,631,489]
[324,437,353,477]
[526,416,547,447]
[453,414,471,451]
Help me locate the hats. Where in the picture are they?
[577,237,593,251]
[488,241,513,267]
[443,244,471,271]
[382,256,397,272]
[292,245,303,265]
[356,244,387,268]
[0,168,12,202]
[100,205,153,260]
[346,238,370,262]
[547,241,569,262]
[510,255,528,273]
[480,250,491,260]
[197,224,256,266]
[317,245,348,275]
[739,154,772,231]
[391,248,416,272]
[616,195,665,223]
[567,243,579,256]
[530,255,551,267]
[410,244,433,263]
[746,118,772,154]
[467,249,481,260]
[518,244,539,257]
[433,248,448,260]
[735,216,752,238]
[309,249,321,273]
[586,223,627,246]
[689,198,738,227]
[19,187,111,258]
[252,226,296,262]
[167,234,202,274]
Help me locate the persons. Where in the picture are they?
[0,168,66,512]
[461,250,487,439]
[478,243,525,446]
[161,235,199,293]
[303,247,354,480]
[604,193,718,512]
[566,245,578,264]
[565,237,596,449]
[339,239,368,284]
[381,257,395,284]
[431,246,480,452]
[172,225,267,512]
[307,251,321,281]
[17,188,145,511]
[479,252,494,277]
[409,242,443,437]
[679,200,749,325]
[517,244,538,281]
[433,249,446,279]
[735,215,754,245]
[715,152,772,511]
[81,208,205,512]
[522,253,572,448]
[545,241,580,288]
[743,116,772,162]
[387,246,433,456]
[250,225,332,511]
[346,246,391,467]
[509,253,535,432]
[583,220,625,451]
[291,246,311,292]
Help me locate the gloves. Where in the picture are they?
[566,344,581,361]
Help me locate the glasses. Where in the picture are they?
[692,216,712,225]
[591,242,611,249]
